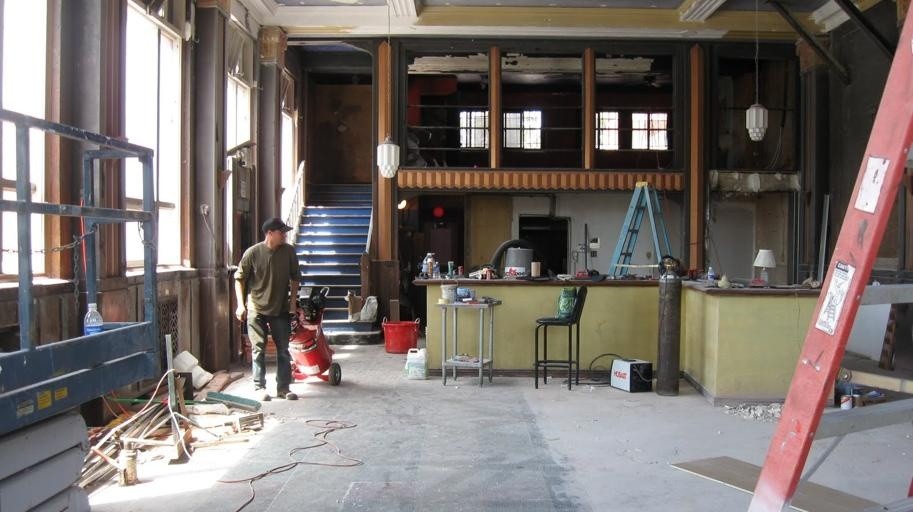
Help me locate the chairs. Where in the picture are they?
[534,285,587,390]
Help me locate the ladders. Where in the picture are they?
[607,181,673,275]
[747,1,912,512]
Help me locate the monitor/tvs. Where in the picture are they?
[518,214,570,282]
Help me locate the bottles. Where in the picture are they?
[435,262,439,279]
[426,253,432,278]
[432,264,436,279]
[707,267,714,281]
[422,256,428,279]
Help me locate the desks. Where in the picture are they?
[681,280,835,409]
[412,277,682,395]
[435,300,501,388]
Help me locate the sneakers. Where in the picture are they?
[253,387,272,401]
[278,388,298,399]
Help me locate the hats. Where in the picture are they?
[261,218,293,234]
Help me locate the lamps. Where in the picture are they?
[397,200,406,209]
[376,3,400,178]
[745,1,767,141]
[752,249,775,282]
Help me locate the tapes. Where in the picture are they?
[463,298,473,302]
[439,299,450,305]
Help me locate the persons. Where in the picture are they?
[233,218,303,400]
